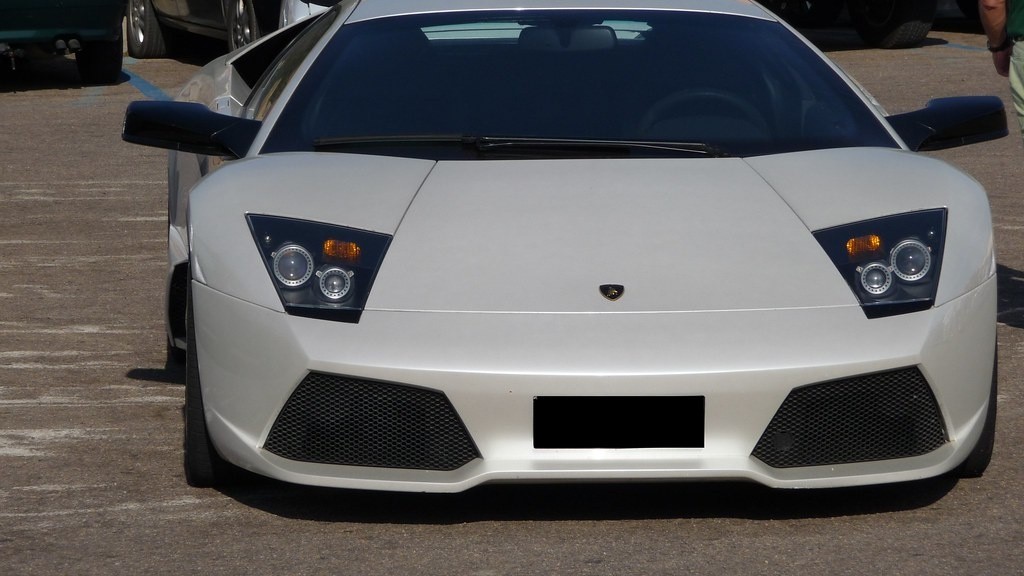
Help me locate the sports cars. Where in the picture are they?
[119,0,999,492]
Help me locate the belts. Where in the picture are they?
[1009,36,1024,41]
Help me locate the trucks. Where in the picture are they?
[0,0,127,81]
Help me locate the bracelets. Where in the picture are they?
[987,36,1011,52]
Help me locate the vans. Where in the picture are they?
[122,0,651,75]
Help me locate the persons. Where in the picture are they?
[977,0,1024,157]
